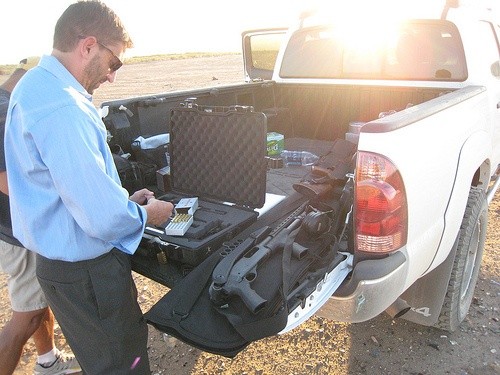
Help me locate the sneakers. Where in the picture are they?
[32,351,82,374]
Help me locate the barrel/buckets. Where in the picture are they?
[266,132,284,157]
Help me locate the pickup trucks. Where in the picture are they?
[101,2,500,334]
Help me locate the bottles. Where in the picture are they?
[279,149,321,166]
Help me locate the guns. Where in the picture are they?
[208,198,315,316]
[293,155,354,198]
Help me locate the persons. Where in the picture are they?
[3,0,175,375]
[0,53,87,375]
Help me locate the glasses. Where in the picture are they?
[79,31,125,74]
